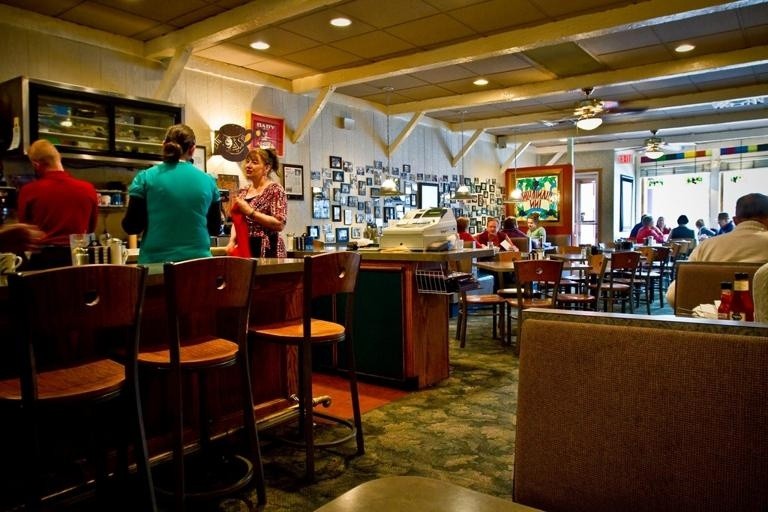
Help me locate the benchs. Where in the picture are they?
[499,303,767,512]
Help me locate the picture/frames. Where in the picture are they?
[189,143,207,175]
[312,471,548,512]
[618,174,637,235]
[249,111,286,160]
[508,167,565,227]
[279,154,508,244]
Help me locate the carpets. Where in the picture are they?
[298,371,412,431]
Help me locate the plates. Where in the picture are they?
[0,73,190,172]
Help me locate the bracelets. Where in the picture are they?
[75,108,97,118]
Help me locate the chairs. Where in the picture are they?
[432,206,767,355]
[0,261,162,512]
[249,242,371,476]
[123,252,274,512]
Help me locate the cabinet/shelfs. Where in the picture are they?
[0,73,190,172]
[97,193,102,205]
[107,238,125,265]
[717,280,733,320]
[76,254,90,266]
[117,109,141,124]
[614,236,632,251]
[286,231,313,251]
[643,236,657,246]
[580,246,591,257]
[528,251,544,260]
[128,234,138,250]
[732,272,755,323]
[539,234,544,249]
[101,195,111,207]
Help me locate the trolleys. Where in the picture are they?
[606,130,699,161]
[557,84,649,124]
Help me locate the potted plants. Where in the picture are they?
[189,143,207,175]
[508,167,565,227]
[618,174,637,235]
[279,154,508,244]
[249,111,286,160]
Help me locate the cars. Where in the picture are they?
[68,232,89,267]
[112,192,122,204]
[487,241,493,250]
[0,252,23,274]
[457,239,464,250]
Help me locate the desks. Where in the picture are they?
[312,471,548,512]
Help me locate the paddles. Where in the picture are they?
[298,371,412,431]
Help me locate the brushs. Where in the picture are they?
[1,187,19,226]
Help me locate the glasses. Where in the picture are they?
[373,86,406,198]
[449,108,475,200]
[575,109,605,134]
[504,123,530,203]
[645,142,665,161]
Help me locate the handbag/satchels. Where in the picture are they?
[248,207,256,220]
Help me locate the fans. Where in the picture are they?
[606,130,699,161]
[557,84,649,124]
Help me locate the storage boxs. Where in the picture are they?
[499,303,767,512]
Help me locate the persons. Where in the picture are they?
[121,123,222,265]
[15,138,99,266]
[2,221,49,253]
[629,193,768,304]
[227,147,289,257]
[455,215,549,254]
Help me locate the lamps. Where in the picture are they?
[575,109,605,134]
[373,86,406,198]
[0,261,162,512]
[123,252,274,512]
[504,123,530,203]
[449,108,475,200]
[249,242,371,476]
[645,142,665,161]
